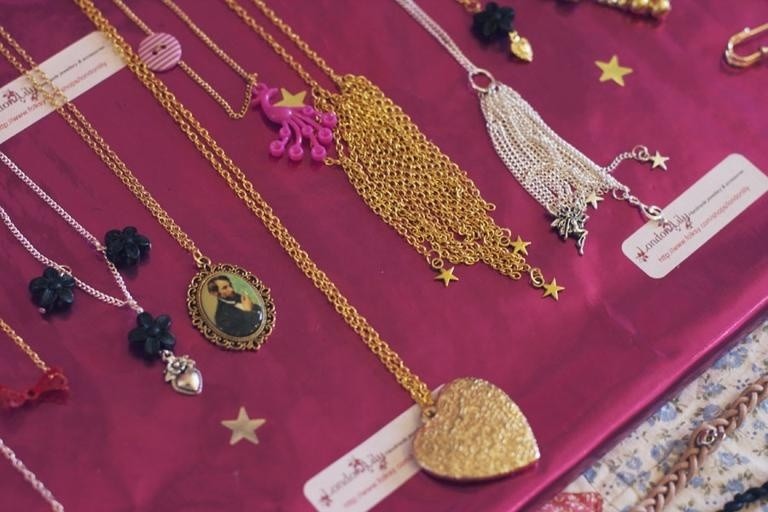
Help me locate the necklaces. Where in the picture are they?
[399,1,671,257]
[113,1,337,163]
[459,2,534,63]
[0,318,71,415]
[225,0,567,305]
[0,26,278,354]
[78,0,542,483]
[0,150,205,397]
